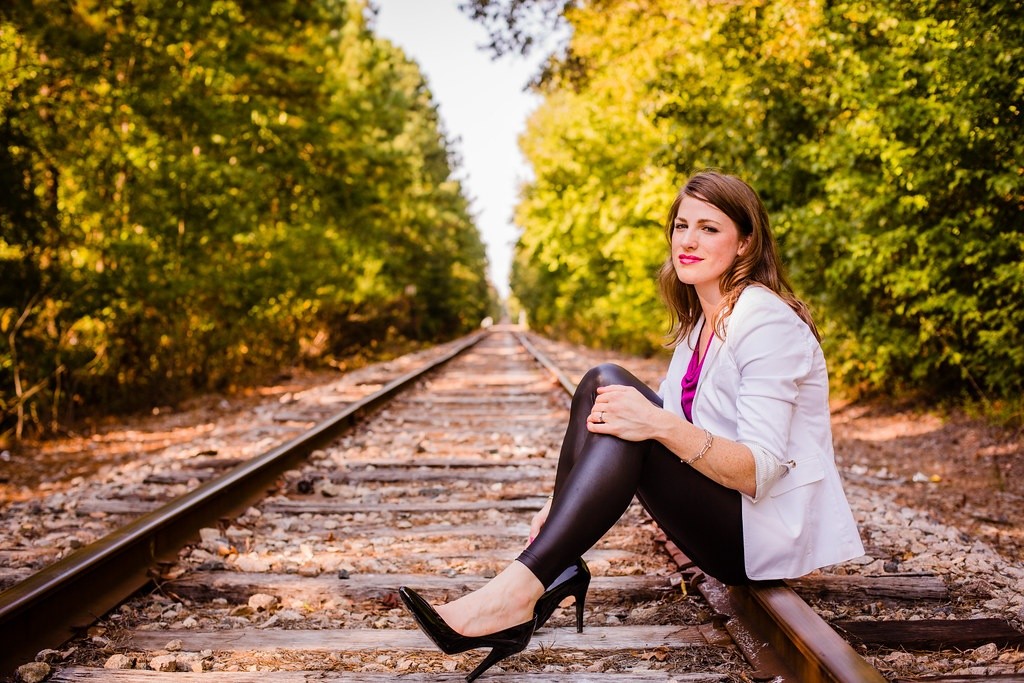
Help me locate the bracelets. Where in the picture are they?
[681,429,713,464]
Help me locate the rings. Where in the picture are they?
[599,411,604,422]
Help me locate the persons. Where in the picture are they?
[399,172,865,683]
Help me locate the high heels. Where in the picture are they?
[398,586,537,682]
[533,556,591,633]
